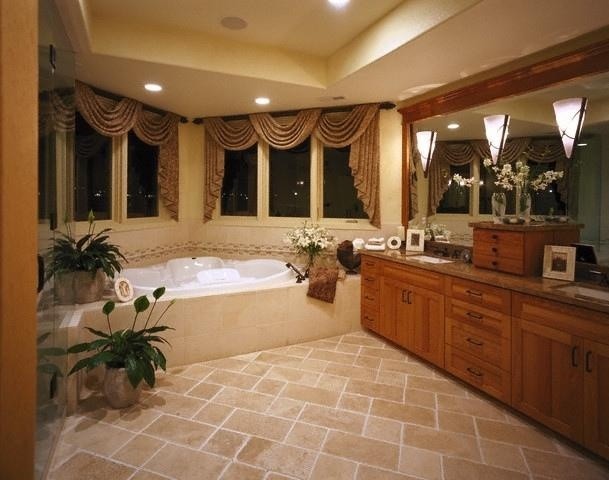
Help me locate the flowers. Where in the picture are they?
[284,220,339,268]
[483,158,530,212]
[452,173,474,189]
[510,161,564,212]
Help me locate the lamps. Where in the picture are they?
[552,97,588,159]
[483,115,510,165]
[416,130,437,179]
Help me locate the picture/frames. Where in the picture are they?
[572,242,599,264]
[542,245,576,282]
[406,229,424,251]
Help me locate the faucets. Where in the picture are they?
[286,261,306,281]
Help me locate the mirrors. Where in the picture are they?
[395,50,609,270]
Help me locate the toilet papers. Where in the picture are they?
[386,236,401,249]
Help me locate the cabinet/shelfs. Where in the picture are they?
[361,254,380,334]
[468,222,585,275]
[380,258,444,370]
[512,289,609,461]
[444,274,511,406]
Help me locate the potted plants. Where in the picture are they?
[41,210,128,305]
[67,287,176,408]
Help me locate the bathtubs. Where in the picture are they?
[114,255,292,299]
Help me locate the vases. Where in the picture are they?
[516,193,531,221]
[491,193,506,224]
[304,254,315,278]
